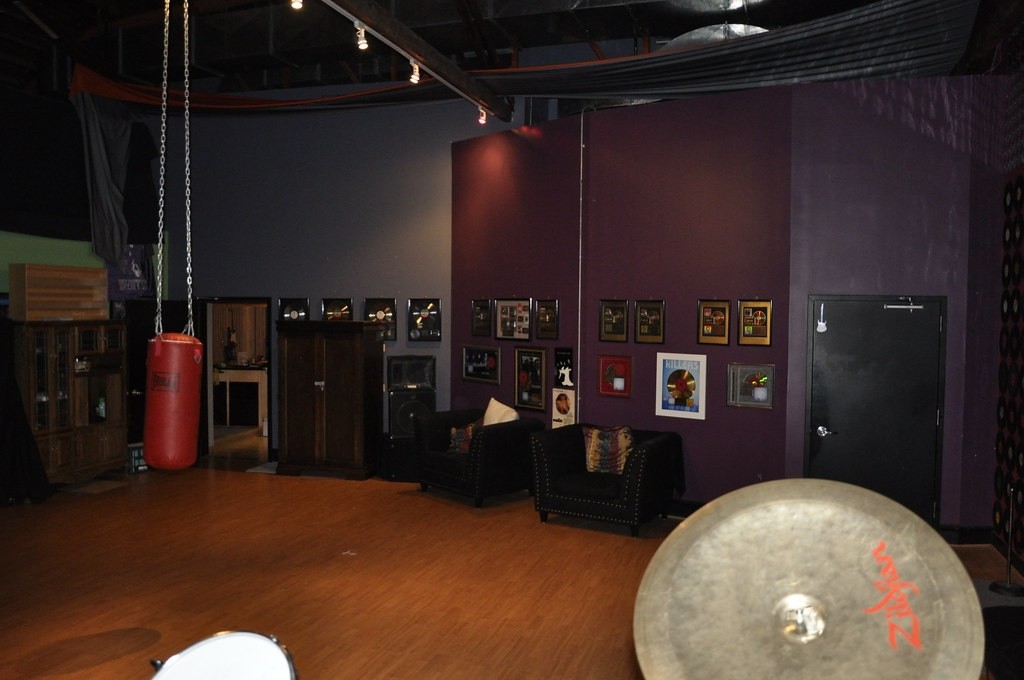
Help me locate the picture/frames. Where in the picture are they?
[494,298,533,341]
[321,297,354,319]
[698,298,731,346]
[363,297,397,341]
[472,300,491,336]
[535,299,559,339]
[599,298,629,343]
[407,298,442,341]
[655,352,706,420]
[278,298,310,318]
[462,344,501,385]
[596,355,632,397]
[738,299,772,346]
[727,362,776,411]
[514,347,546,412]
[634,299,666,344]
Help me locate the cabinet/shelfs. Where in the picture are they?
[13,319,125,487]
[274,319,384,468]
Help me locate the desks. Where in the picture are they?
[221,366,268,429]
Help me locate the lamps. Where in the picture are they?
[356,28,368,50]
[291,0,302,9]
[409,59,419,83]
[477,107,487,124]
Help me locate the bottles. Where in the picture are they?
[262,416,268,436]
[229,328,237,359]
[99,397,105,417]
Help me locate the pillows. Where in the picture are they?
[582,425,634,475]
[482,397,519,426]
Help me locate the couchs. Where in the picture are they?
[412,408,546,512]
[531,422,685,539]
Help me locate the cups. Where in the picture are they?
[237,351,261,365]
